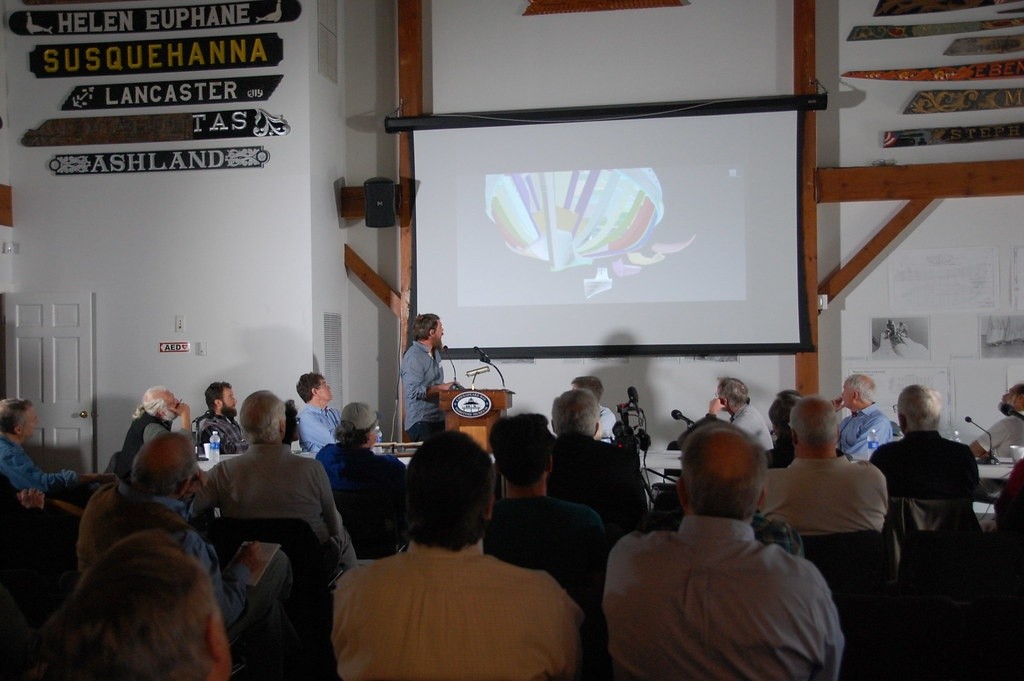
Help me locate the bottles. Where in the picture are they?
[949,431,961,443]
[372,426,383,454]
[867,429,880,461]
[209,430,220,463]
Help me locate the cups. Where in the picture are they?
[203,443,210,458]
[1009,445,1024,469]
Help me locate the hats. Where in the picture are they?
[340,401,377,430]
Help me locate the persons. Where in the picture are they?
[191,391,356,575]
[707,378,773,450]
[766,389,802,468]
[331,431,586,681]
[198,382,248,453]
[831,374,892,461]
[761,395,889,535]
[55,528,233,680]
[0,397,122,680]
[484,413,606,569]
[602,422,844,680]
[401,314,457,442]
[979,460,1024,538]
[115,386,191,484]
[76,433,305,681]
[549,388,649,531]
[315,402,409,560]
[635,508,803,558]
[547,377,616,443]
[296,373,342,453]
[870,384,982,502]
[969,383,1024,457]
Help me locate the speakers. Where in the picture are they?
[363,177,396,228]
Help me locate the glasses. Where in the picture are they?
[892,404,901,414]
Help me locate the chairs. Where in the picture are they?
[0,481,1024,681]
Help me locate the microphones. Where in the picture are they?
[1000,404,1024,420]
[671,410,695,425]
[191,409,215,423]
[627,387,639,415]
[473,346,491,363]
[965,417,1002,465]
[444,345,464,390]
